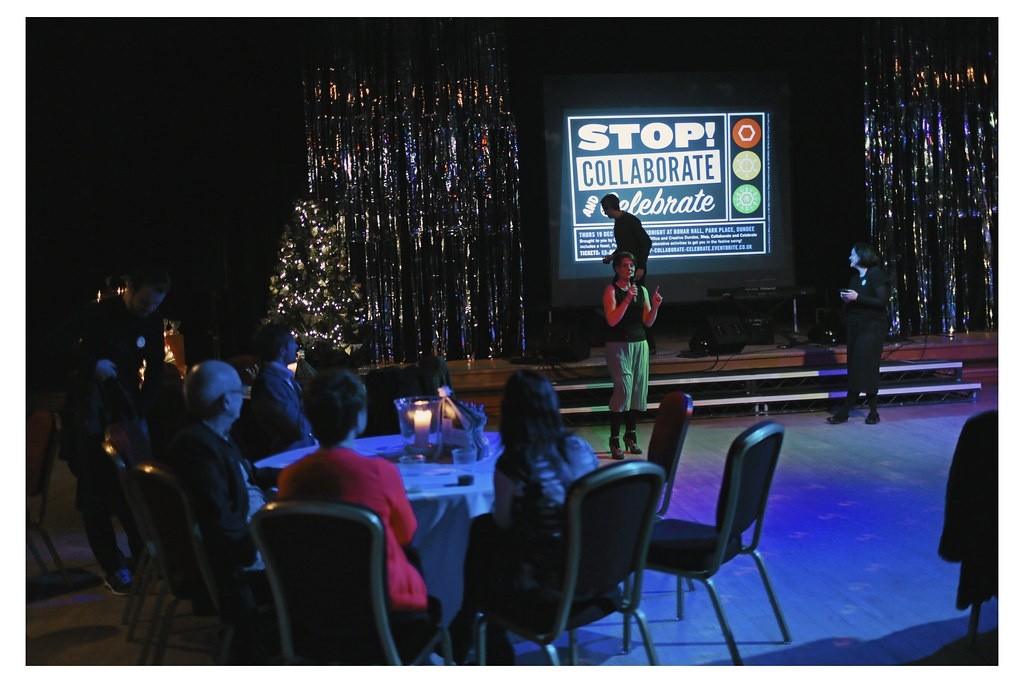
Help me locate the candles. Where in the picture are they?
[414,409,432,447]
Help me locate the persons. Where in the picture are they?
[167,324,599,666]
[62,265,175,597]
[826,241,891,425]
[601,193,657,356]
[601,252,663,460]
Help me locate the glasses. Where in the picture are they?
[229,385,248,395]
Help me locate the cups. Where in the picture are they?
[399,456,425,492]
[452,448,477,486]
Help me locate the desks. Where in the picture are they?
[252,430,505,665]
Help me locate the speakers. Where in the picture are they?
[806,311,847,346]
[688,315,749,355]
[541,320,591,362]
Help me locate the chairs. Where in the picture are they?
[26,402,243,665]
[648,392,692,517]
[622,418,791,665]
[249,499,456,666]
[472,459,667,666]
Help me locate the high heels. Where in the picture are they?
[623,430,642,454]
[609,436,624,459]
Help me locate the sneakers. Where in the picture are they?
[105,567,133,595]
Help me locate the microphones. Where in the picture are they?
[630,276,637,302]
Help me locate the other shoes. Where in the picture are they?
[827,412,849,423]
[866,413,880,423]
